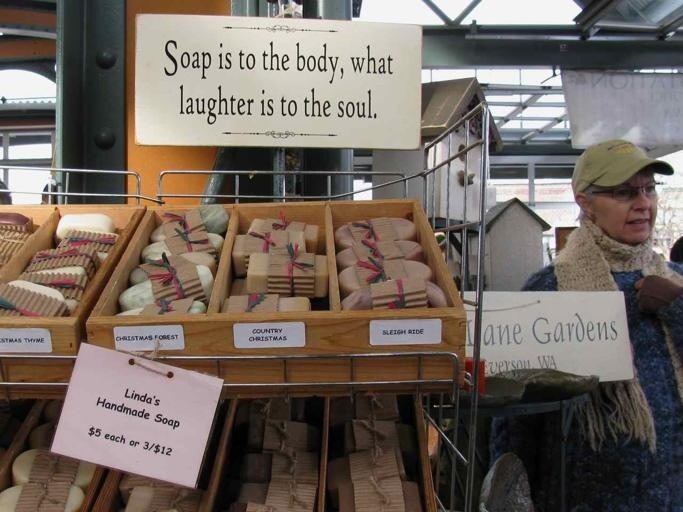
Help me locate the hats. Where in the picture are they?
[572,140,674,197]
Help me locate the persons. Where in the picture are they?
[488,140,683,512]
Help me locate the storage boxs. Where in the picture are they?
[0,392,216,512]
[0,201,144,392]
[86,200,467,385]
[204,385,440,512]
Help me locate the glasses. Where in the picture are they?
[580,181,657,201]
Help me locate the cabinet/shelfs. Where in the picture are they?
[0,100,487,512]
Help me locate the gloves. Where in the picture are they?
[638,275,683,313]
